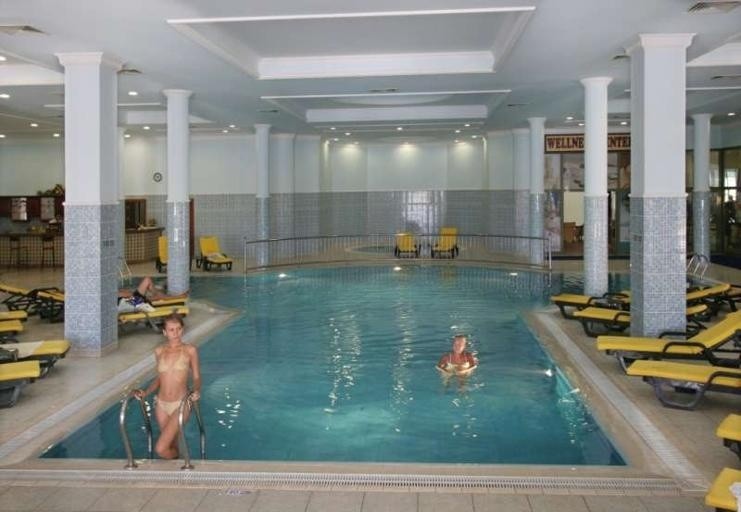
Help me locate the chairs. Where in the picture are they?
[196,235,233,272]
[0,280,73,408]
[8,233,56,272]
[156,236,168,273]
[394,227,461,260]
[116,289,189,335]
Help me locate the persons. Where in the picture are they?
[117,276,190,313]
[436,335,477,394]
[133,313,200,460]
